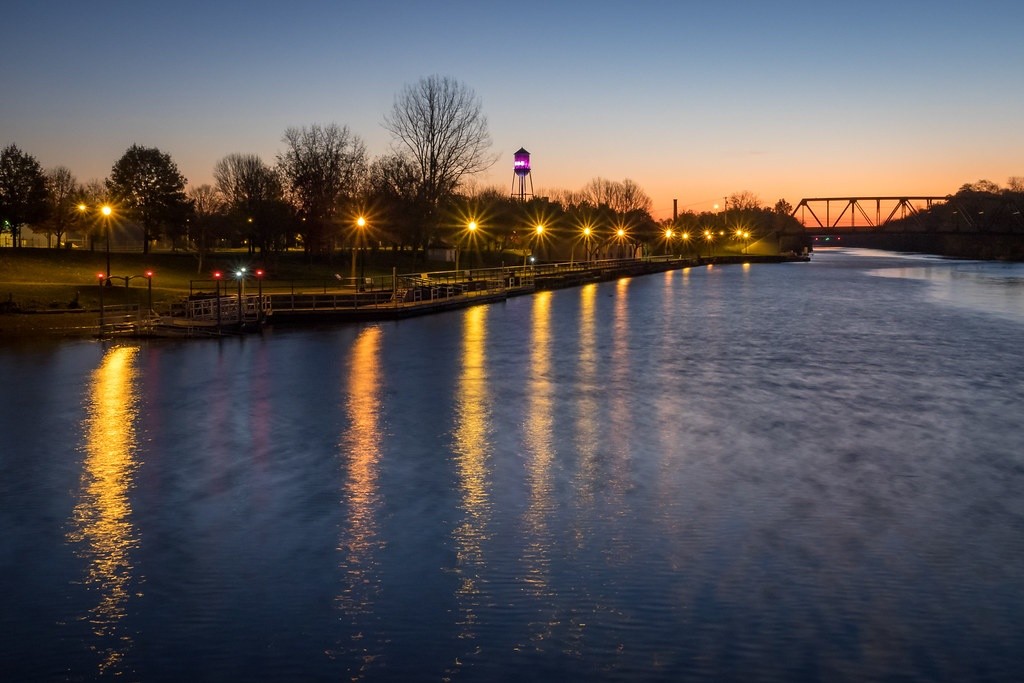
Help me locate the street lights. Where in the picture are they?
[744,232,748,255]
[358,216,366,292]
[707,235,712,256]
[683,234,688,255]
[584,227,590,260]
[102,205,113,290]
[666,230,671,254]
[469,220,476,281]
[618,230,623,259]
[536,225,544,273]
